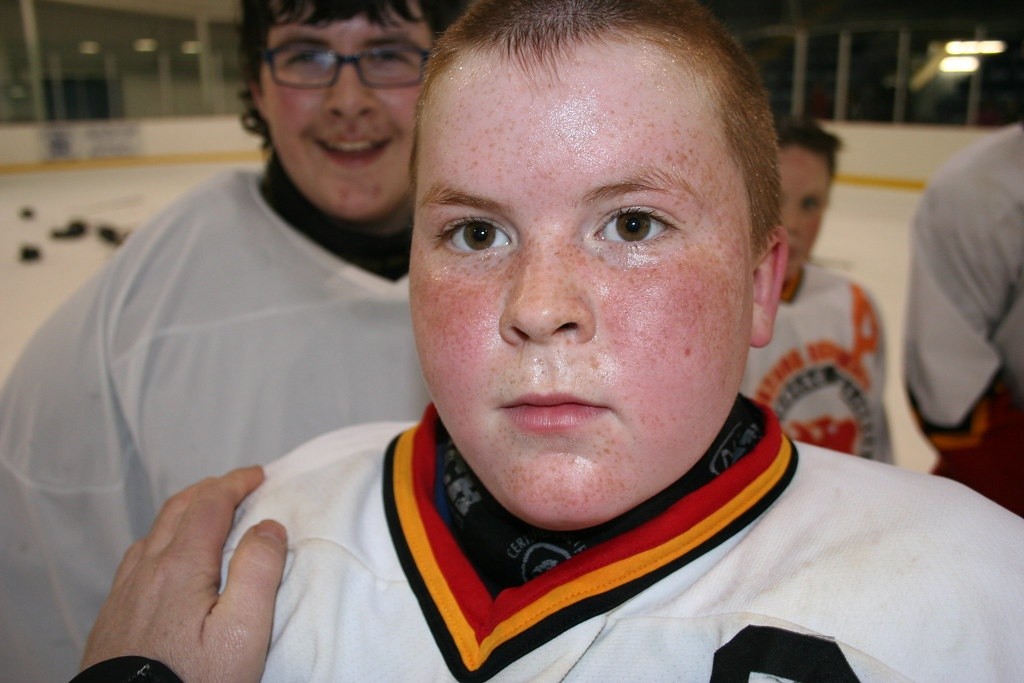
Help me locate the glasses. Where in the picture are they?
[250,40,431,88]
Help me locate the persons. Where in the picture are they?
[221,0,1024,683]
[904,111,1024,519]
[1,1,446,682]
[738,108,893,464]
[65,463,288,683]
